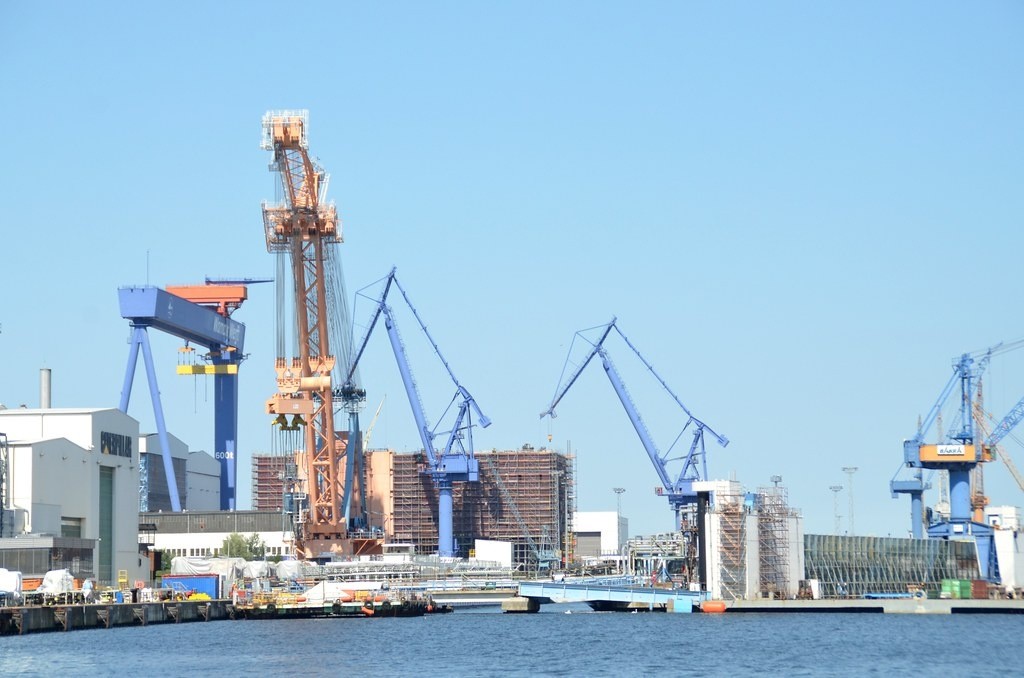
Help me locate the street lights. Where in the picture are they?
[613,487,625,554]
[829,485,843,535]
[843,467,858,535]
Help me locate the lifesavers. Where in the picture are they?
[267,604,276,612]
[365,601,374,609]
[382,601,391,610]
[402,600,411,609]
